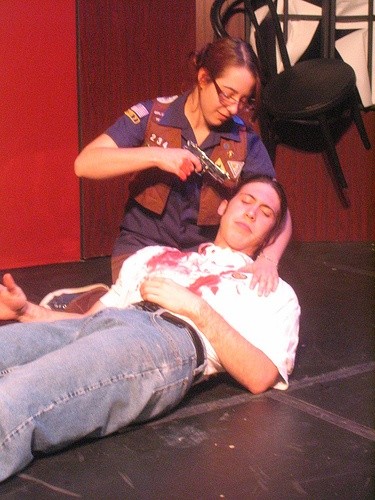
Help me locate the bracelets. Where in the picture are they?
[259,253,277,266]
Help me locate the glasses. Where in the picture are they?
[209,76,254,113]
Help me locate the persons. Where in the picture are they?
[38,37,293,314]
[0,175,302,482]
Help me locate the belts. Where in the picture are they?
[130,301,205,384]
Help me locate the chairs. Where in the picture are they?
[210,0,371,188]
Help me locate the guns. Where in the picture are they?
[181,138,228,185]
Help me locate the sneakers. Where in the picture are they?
[39,281,109,312]
